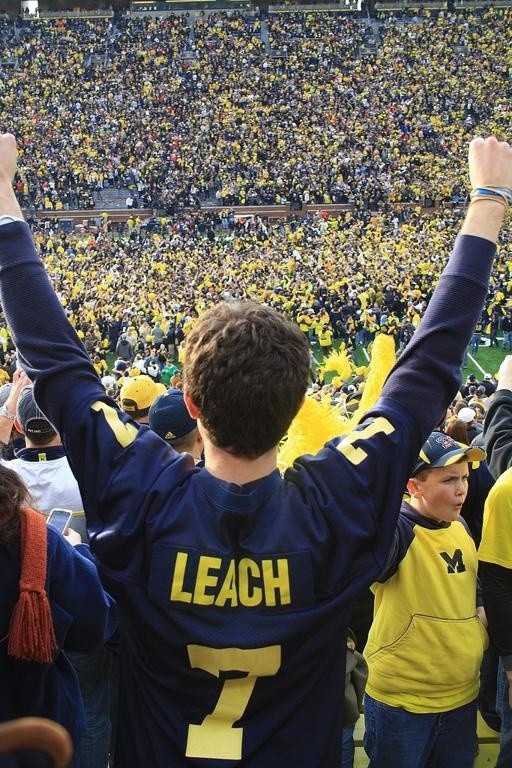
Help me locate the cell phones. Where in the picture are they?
[45,508,73,536]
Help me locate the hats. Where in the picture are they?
[456,407,475,423]
[100,369,169,411]
[410,429,489,479]
[466,372,492,383]
[0,381,58,435]
[146,387,198,441]
